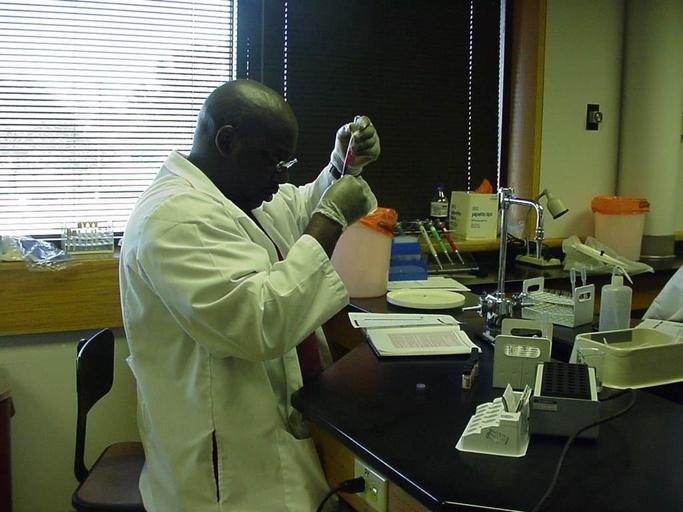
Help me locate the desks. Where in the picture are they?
[291,250,683,512]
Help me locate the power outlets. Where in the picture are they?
[354,458,389,512]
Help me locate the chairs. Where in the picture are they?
[71,328,146,512]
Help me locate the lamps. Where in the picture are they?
[515,188,570,267]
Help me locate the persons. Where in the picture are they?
[114,78,391,512]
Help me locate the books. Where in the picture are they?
[354,313,483,359]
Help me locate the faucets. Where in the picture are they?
[488,188,545,320]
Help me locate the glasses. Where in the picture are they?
[235,129,298,173]
